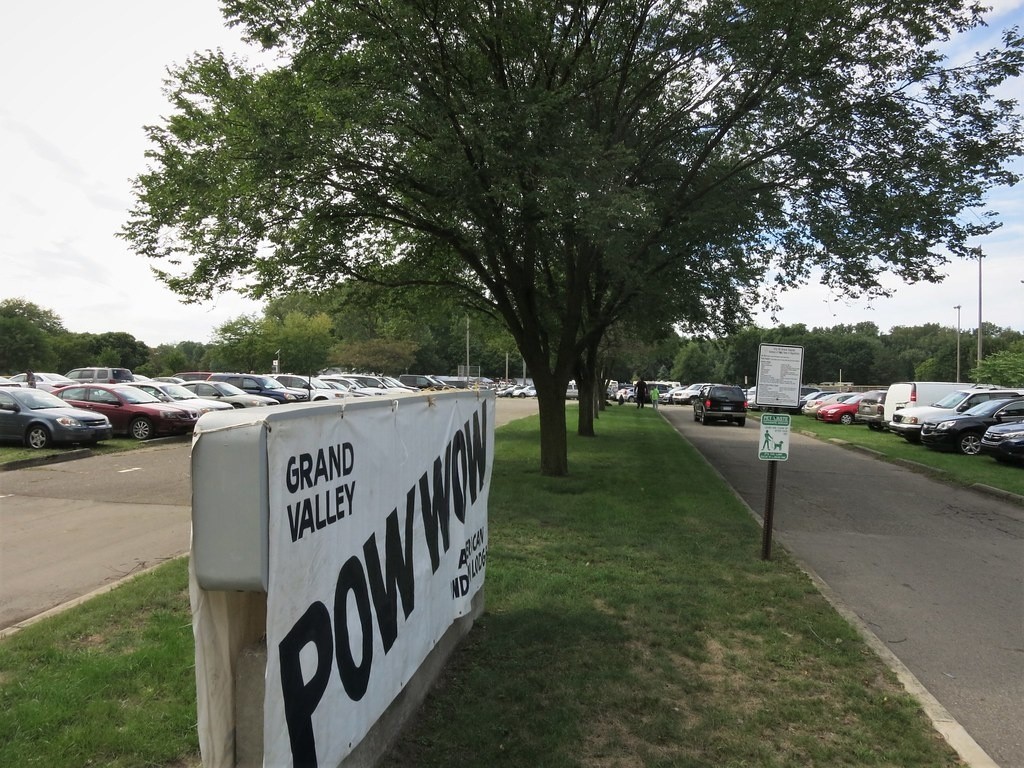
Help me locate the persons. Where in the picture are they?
[650,386,659,410]
[26,369,36,389]
[634,376,648,409]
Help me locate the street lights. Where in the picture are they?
[973,245,984,384]
[952,305,963,383]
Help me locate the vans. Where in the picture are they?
[881,381,1008,434]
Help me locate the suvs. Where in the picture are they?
[692,385,749,426]
[889,385,1024,445]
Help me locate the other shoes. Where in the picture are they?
[641,406,645,408]
[637,405,639,410]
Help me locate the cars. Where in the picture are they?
[0,385,113,451]
[980,419,1024,468]
[1,364,886,442]
[920,397,1024,456]
